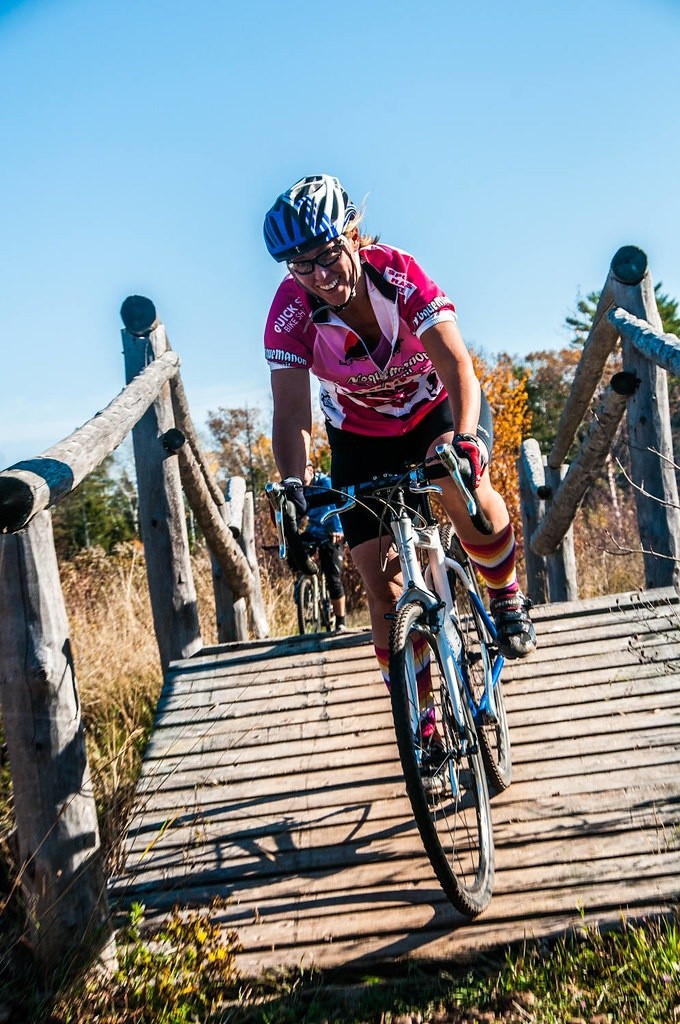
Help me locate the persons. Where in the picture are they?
[294,460,346,634]
[263,175,537,804]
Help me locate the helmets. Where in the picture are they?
[263,175,359,263]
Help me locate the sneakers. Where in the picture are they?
[418,742,448,795]
[490,596,536,659]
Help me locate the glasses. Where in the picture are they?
[286,236,345,275]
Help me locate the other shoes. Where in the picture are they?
[336,624,347,636]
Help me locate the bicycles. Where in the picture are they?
[263,442,514,916]
[287,535,337,635]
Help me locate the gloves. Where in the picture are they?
[268,476,307,528]
[452,434,486,490]
